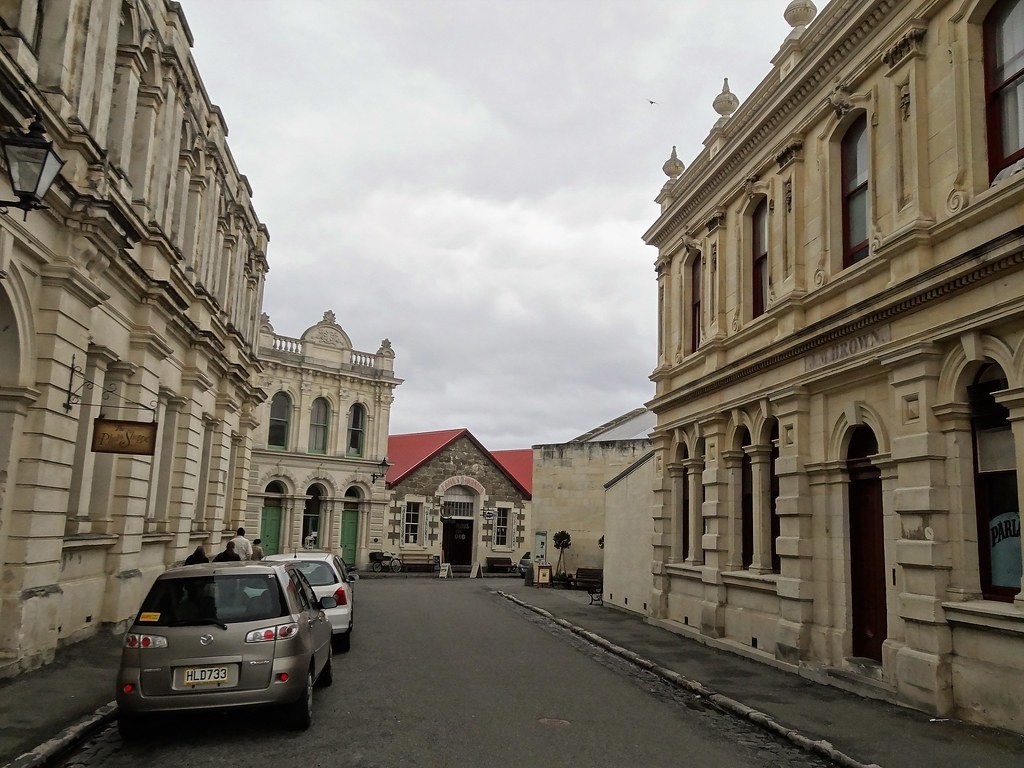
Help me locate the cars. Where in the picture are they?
[517,552,531,576]
[115,559,336,743]
[261,553,360,655]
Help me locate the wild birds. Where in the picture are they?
[646,99,659,105]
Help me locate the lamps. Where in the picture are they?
[370,457,389,484]
[0,112,67,223]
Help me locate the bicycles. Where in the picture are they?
[372,549,402,573]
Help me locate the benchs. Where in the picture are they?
[398,553,436,572]
[567,568,602,590]
[587,574,602,606]
[486,557,511,573]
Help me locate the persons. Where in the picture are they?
[182,546,209,566]
[211,541,242,562]
[230,528,253,561]
[251,539,264,561]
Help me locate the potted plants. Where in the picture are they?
[552,531,571,589]
